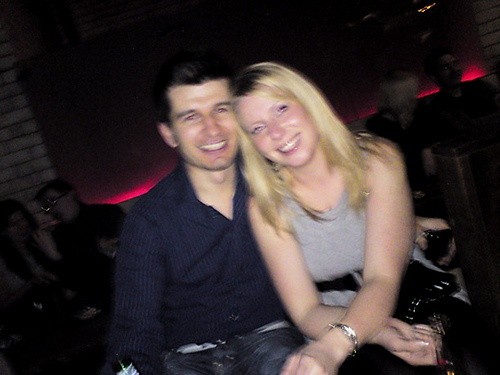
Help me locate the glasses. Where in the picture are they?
[38,194,66,215]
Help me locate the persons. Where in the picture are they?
[231,62,475,375]
[365,53,499,243]
[0,199,84,310]
[31,181,128,294]
[106,62,457,375]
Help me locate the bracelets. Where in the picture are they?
[326,322,359,359]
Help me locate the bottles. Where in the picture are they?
[428,313,456,375]
[112,356,139,375]
